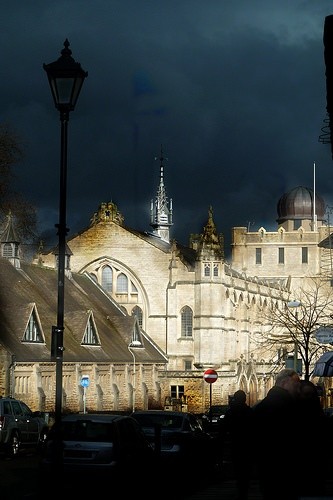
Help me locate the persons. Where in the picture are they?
[222,370,333,500]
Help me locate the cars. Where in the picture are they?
[0,394,226,468]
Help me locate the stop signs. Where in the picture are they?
[203,369,218,383]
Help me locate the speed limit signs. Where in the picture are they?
[80,379,90,387]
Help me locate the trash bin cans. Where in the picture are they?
[182,402,188,413]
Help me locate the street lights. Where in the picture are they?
[286,301,301,372]
[127,340,142,411]
[43,37,89,423]
